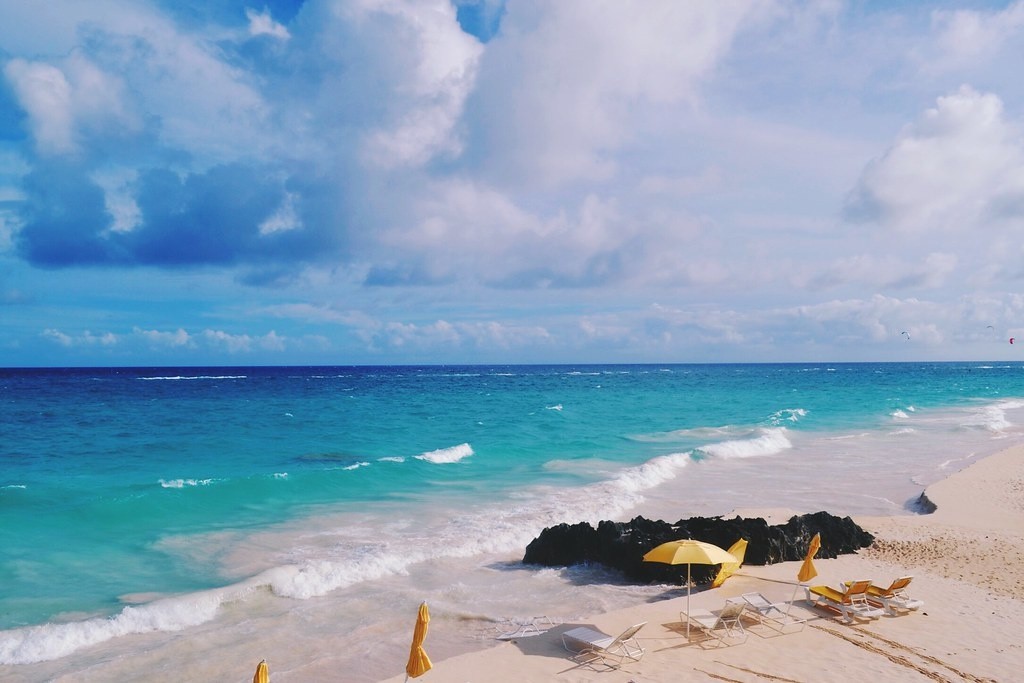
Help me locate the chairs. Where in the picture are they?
[497,575,923,671]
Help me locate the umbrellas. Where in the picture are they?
[714,534,750,586]
[404,600,432,683]
[785,532,822,619]
[253,659,269,683]
[643,537,737,638]
